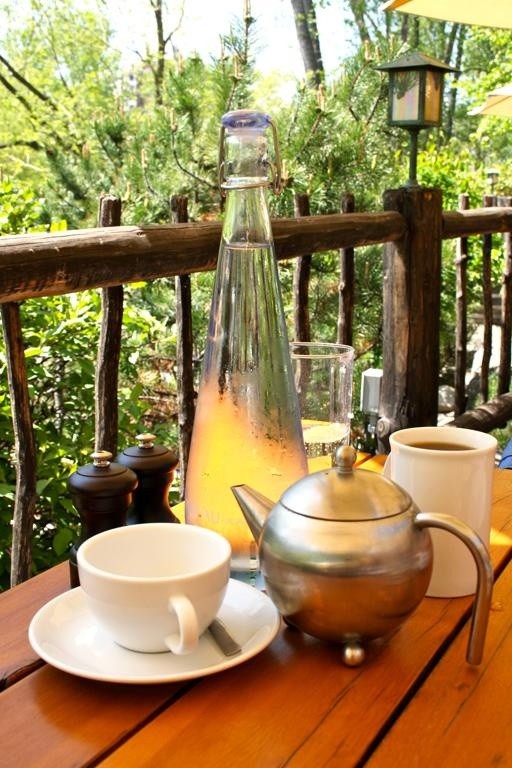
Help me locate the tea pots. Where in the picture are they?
[228,443,496,672]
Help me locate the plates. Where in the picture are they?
[26,577,281,685]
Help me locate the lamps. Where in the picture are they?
[372,51,462,189]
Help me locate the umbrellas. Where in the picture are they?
[378,0,510,32]
[465,78,510,121]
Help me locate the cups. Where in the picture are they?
[379,425,499,601]
[282,339,355,481]
[71,519,233,657]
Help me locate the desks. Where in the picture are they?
[0,448,512,767]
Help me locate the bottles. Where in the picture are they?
[114,432,185,526]
[63,449,139,591]
[180,108,315,595]
